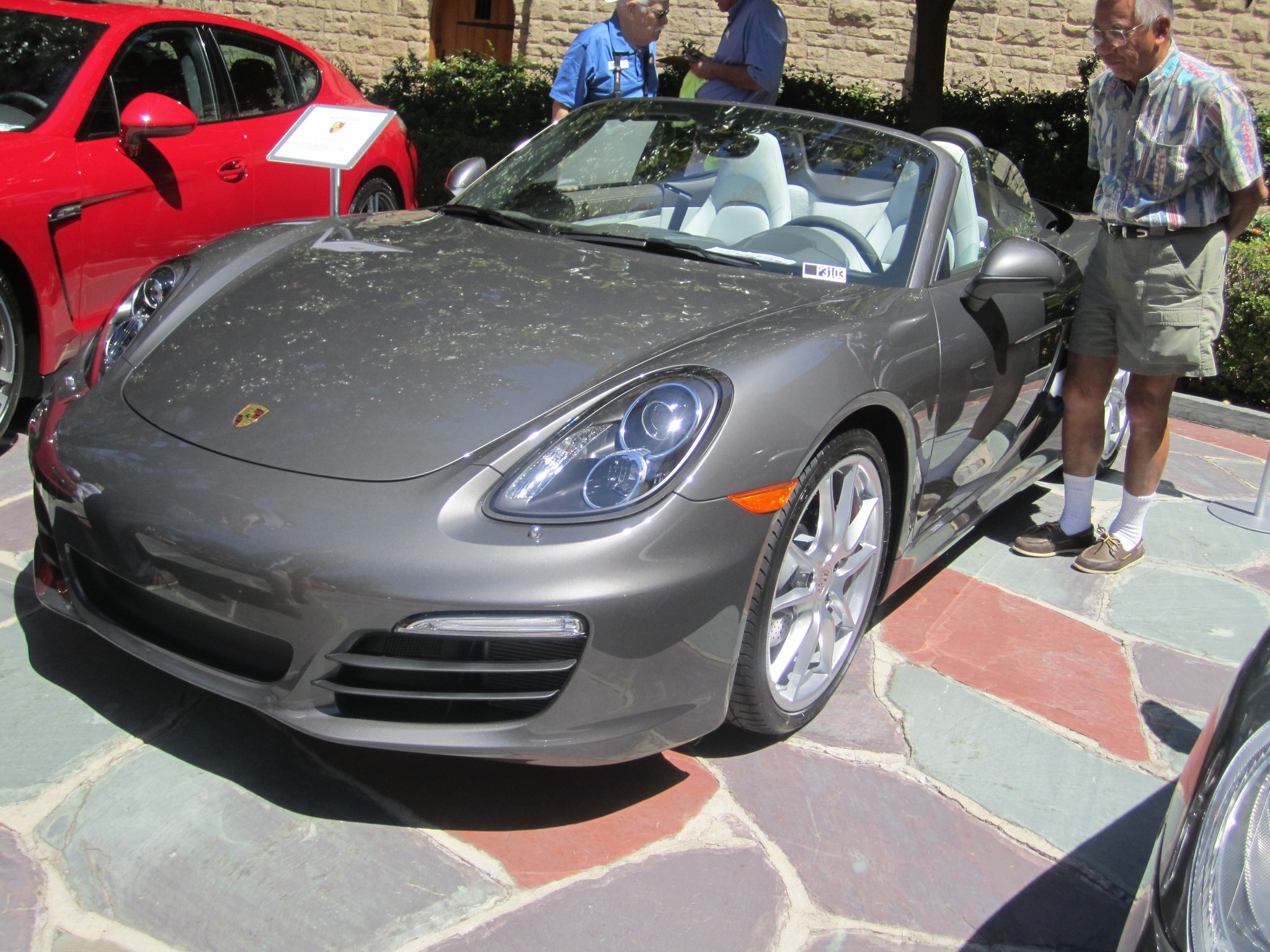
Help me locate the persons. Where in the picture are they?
[1011,0,1268,574]
[681,0,788,177]
[549,0,669,191]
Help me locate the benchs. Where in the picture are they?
[230,58,285,115]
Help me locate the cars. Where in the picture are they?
[1115,624,1270,952]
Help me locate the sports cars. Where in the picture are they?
[27,95,1129,771]
[1,0,417,453]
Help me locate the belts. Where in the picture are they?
[1101,217,1194,238]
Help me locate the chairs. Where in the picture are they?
[100,51,190,130]
[686,132,791,245]
[850,141,980,274]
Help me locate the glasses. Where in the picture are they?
[1084,14,1159,47]
[635,2,670,20]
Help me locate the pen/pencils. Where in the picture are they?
[690,43,705,56]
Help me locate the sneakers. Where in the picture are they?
[1072,524,1147,575]
[1013,521,1095,557]
